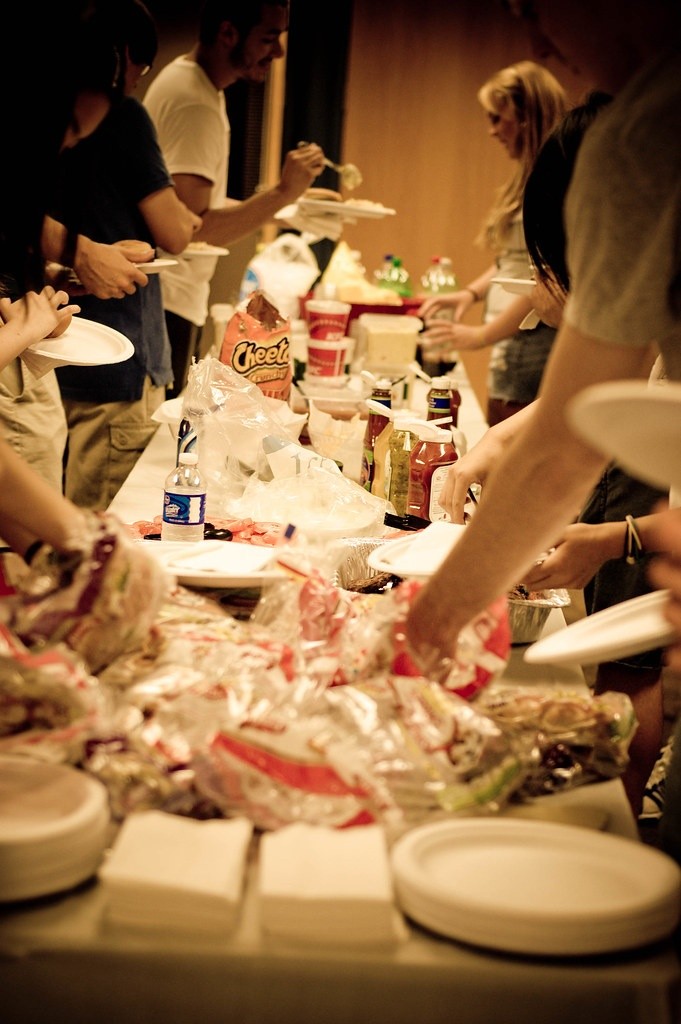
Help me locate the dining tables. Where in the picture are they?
[0,347,681,1024]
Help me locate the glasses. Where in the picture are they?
[139,63,152,75]
[488,113,502,127]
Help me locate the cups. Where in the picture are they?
[305,299,352,341]
[359,311,423,380]
[307,338,348,377]
[419,340,454,376]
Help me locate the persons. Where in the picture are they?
[419,61,571,423]
[136,0,325,399]
[392,0,680,821]
[1,0,203,589]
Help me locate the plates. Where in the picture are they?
[1,754,113,901]
[298,198,398,220]
[180,243,231,259]
[523,590,679,668]
[392,815,681,953]
[67,258,178,285]
[363,529,437,581]
[491,278,536,297]
[565,382,681,497]
[24,314,136,367]
[137,540,289,588]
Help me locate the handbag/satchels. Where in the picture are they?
[238,232,319,319]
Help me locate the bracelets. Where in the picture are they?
[24,539,43,566]
[466,283,483,306]
[52,267,71,290]
[625,514,643,566]
[58,224,80,270]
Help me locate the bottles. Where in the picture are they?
[424,376,456,450]
[159,452,205,540]
[360,381,392,494]
[371,411,414,501]
[385,417,419,526]
[375,254,412,298]
[420,255,459,296]
[403,428,459,529]
[450,380,461,428]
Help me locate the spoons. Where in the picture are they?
[298,141,363,189]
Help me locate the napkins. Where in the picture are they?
[95,809,257,944]
[254,803,413,950]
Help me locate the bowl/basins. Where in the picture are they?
[162,398,182,445]
[291,376,372,435]
